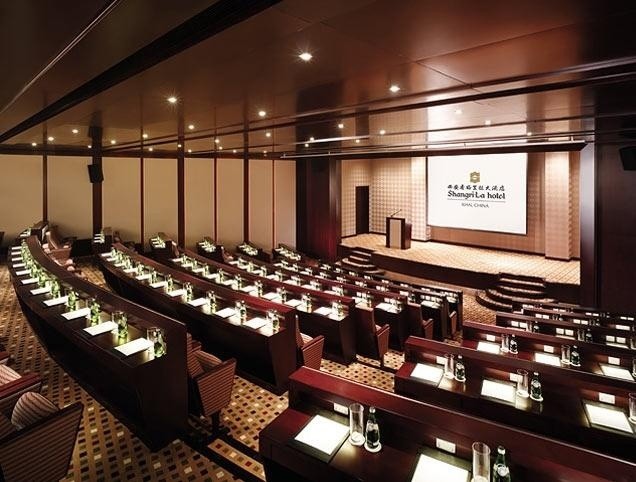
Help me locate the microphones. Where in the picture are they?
[391,209,402,217]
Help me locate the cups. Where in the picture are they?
[349,402,364,443]
[631,339,636,350]
[472,442,491,482]
[632,359,636,377]
[502,334,509,351]
[526,320,532,332]
[562,345,571,364]
[628,392,636,425]
[517,369,529,395]
[444,354,454,376]
[578,329,585,341]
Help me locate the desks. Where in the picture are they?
[8,219,635,482]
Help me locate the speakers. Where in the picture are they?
[620,145,636,171]
[87,164,104,183]
[311,160,325,172]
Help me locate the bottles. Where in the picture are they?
[455,355,465,380]
[493,446,511,482]
[364,405,380,449]
[20,223,400,344]
[531,371,541,399]
[571,345,580,365]
[534,321,539,333]
[510,334,517,353]
[539,303,611,328]
[586,328,593,343]
